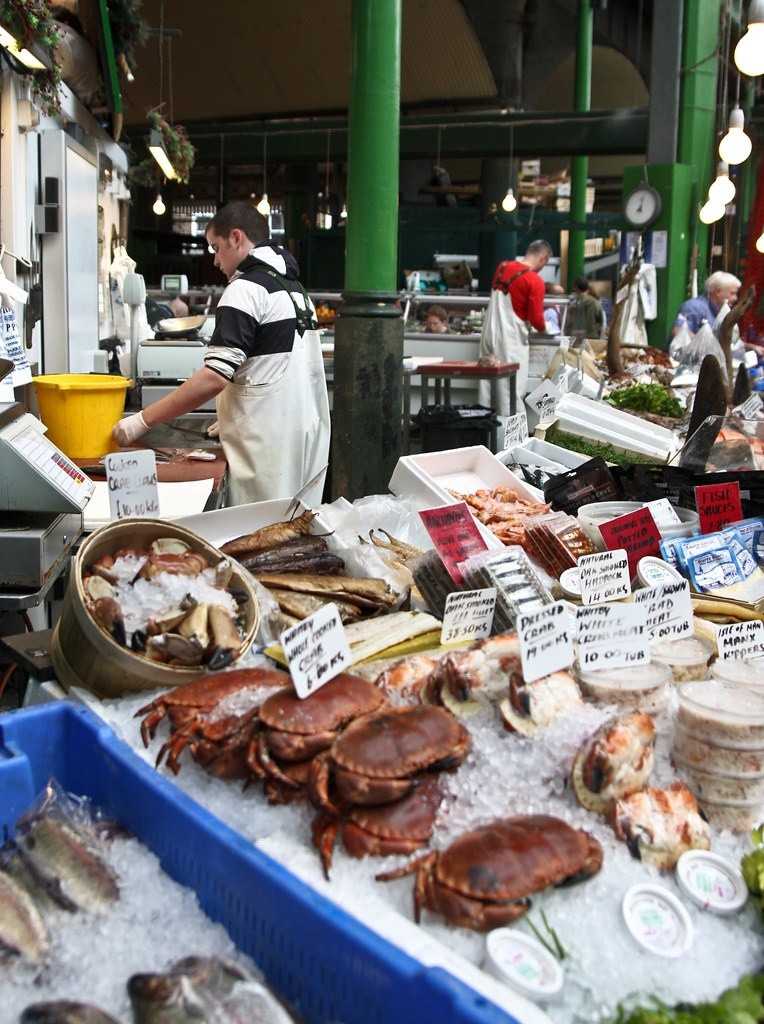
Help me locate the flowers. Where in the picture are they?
[0,0,68,118]
[147,110,195,184]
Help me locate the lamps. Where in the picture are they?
[138,125,178,180]
[0,15,52,70]
[314,129,334,230]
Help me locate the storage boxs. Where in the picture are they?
[167,392,681,612]
[0,700,521,1023]
[579,338,609,360]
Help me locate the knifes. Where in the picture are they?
[172,425,220,444]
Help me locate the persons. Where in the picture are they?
[431,167,458,207]
[478,240,552,418]
[422,305,458,334]
[665,271,742,354]
[156,299,188,319]
[544,275,607,347]
[111,200,331,508]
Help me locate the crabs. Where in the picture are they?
[80,538,712,933]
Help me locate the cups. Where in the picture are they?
[481,927,562,1004]
[674,849,749,915]
[621,883,694,957]
[559,566,582,599]
[629,556,683,592]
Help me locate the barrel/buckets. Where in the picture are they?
[33,374,132,467]
[576,501,700,553]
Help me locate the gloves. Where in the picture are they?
[113,410,151,447]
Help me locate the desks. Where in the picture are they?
[417,359,520,455]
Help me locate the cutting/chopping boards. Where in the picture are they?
[417,361,519,375]
[69,449,227,494]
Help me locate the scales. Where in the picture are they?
[623,163,662,257]
[0,359,97,512]
[138,315,210,381]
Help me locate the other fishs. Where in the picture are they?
[0,808,292,1023]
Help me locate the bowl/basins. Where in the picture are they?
[573,633,715,705]
[670,661,764,831]
